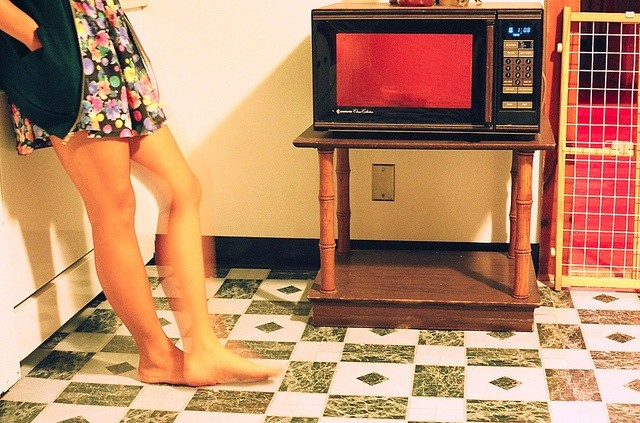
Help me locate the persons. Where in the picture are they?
[0,0,278,386]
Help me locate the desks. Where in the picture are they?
[293,116,555,332]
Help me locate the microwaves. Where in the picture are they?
[311,1,546,140]
